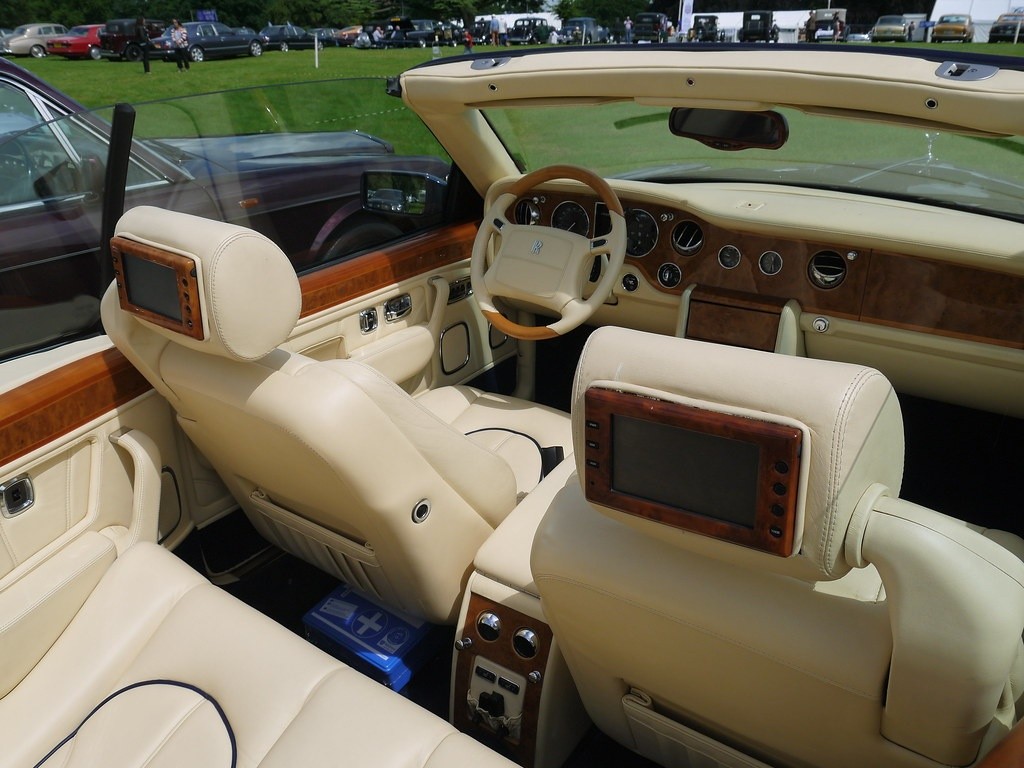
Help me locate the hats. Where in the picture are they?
[461,28,468,32]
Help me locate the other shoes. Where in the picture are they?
[178,68,182,72]
[185,68,189,72]
[145,72,152,75]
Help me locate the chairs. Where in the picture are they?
[100,208,577,648]
[532,323,1024,768]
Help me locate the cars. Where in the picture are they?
[0,54,398,365]
[687,15,726,42]
[97,18,165,62]
[872,15,910,42]
[46,24,106,60]
[631,12,668,43]
[560,17,610,44]
[469,20,493,45]
[736,10,781,43]
[230,15,466,52]
[507,17,556,44]
[139,21,272,61]
[0,23,70,58]
[930,13,975,42]
[815,8,847,43]
[988,6,1024,43]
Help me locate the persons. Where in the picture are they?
[624,16,633,44]
[373,26,387,49]
[807,12,817,43]
[907,21,915,42]
[462,28,475,54]
[598,26,610,44]
[548,27,583,44]
[392,25,406,48]
[134,15,152,74]
[613,17,622,43]
[831,12,840,44]
[489,13,508,47]
[171,17,191,73]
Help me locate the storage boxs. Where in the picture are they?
[302,581,430,696]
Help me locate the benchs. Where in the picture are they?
[0,544,536,768]
